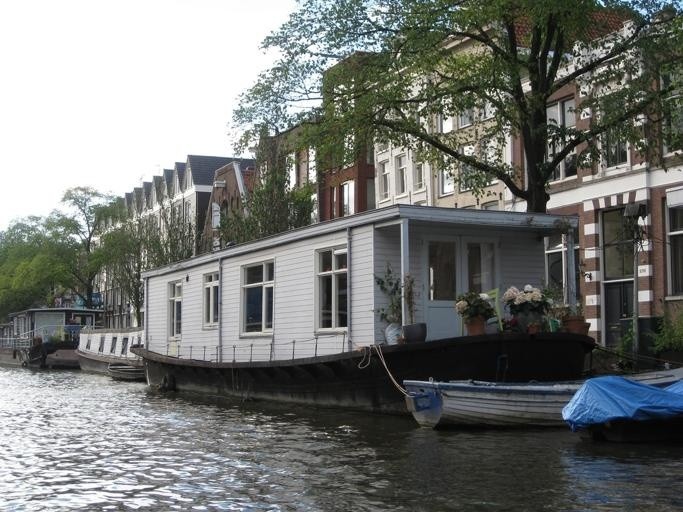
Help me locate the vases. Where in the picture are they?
[465,322,486,336]
[525,323,542,334]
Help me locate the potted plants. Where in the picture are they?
[545,285,591,335]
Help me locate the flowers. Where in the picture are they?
[453,292,497,322]
[502,284,552,322]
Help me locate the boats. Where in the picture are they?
[0,308,104,369]
